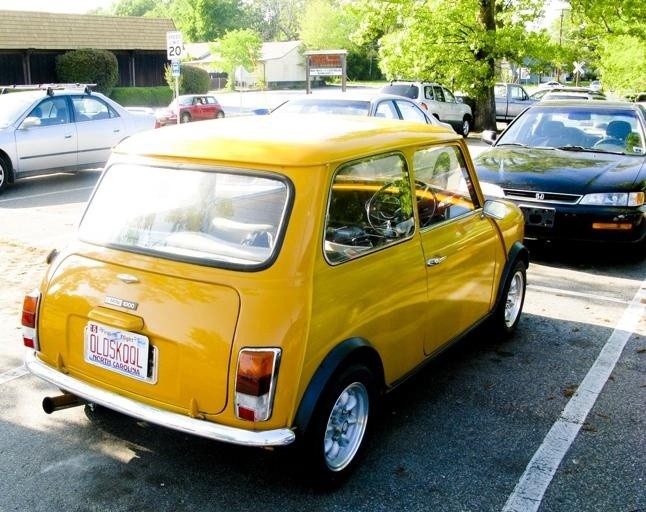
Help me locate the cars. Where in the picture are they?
[2,81,156,201]
[17,107,539,479]
[465,102,646,266]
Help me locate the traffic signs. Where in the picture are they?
[167,31,185,61]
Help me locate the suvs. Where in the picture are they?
[152,93,225,129]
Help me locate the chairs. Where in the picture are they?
[210,217,275,247]
[603,120,631,147]
[57,108,66,123]
[534,122,565,148]
[31,107,42,119]
